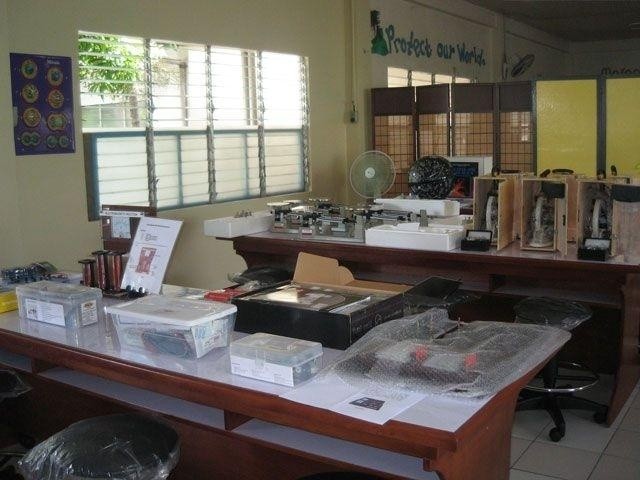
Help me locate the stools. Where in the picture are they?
[11,412,183,480]
[514,296,601,441]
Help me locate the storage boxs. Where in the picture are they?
[15,281,102,328]
[233,279,404,349]
[103,294,236,358]
[578,238,612,261]
[461,230,493,251]
[98,204,157,251]
[230,332,323,385]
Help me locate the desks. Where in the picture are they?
[216,201,640,427]
[1,270,570,480]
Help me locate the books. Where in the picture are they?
[120,217,185,296]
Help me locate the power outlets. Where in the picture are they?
[349,112,358,122]
[503,62,509,80]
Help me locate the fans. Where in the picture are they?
[349,150,396,211]
[509,52,535,77]
